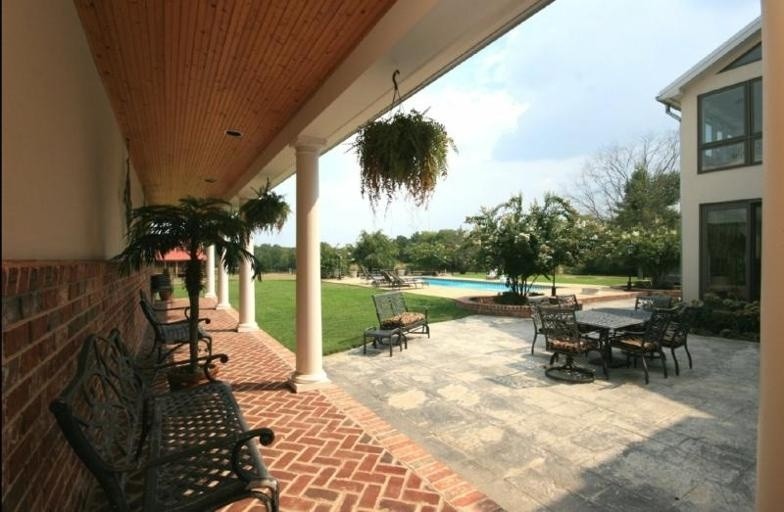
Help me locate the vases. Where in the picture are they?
[161,289,170,301]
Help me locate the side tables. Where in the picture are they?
[363,326,402,356]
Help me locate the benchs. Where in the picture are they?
[48,327,280,512]
[138,285,212,365]
[371,291,430,349]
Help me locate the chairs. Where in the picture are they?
[527,293,692,384]
[381,270,428,291]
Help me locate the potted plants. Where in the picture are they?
[241,180,290,232]
[350,110,456,207]
[107,178,289,392]
